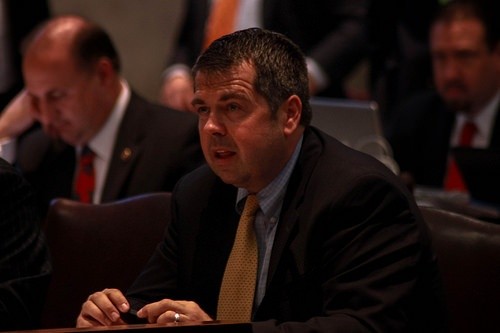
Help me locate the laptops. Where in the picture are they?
[308,99,385,158]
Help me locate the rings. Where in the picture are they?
[173,310,180,322]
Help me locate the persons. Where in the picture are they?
[74,27,453,333]
[0,15,207,207]
[389,0,500,210]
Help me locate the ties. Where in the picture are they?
[217,193,259,325]
[446,120,479,194]
[73,142,97,203]
[202,0,243,50]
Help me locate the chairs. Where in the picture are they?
[43,192,500,333]
